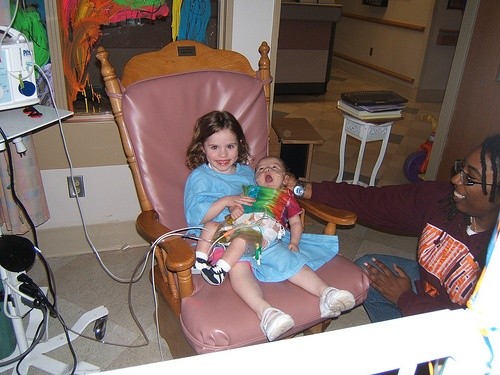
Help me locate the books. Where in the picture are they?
[337,91,408,119]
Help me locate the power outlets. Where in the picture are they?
[67,176,85,198]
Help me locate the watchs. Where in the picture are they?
[293,180,305,199]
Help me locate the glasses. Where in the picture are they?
[454,159,493,186]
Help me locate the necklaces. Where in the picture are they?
[474,217,486,232]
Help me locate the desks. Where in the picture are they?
[337,114,391,187]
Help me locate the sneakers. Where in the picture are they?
[190,257,213,275]
[201,263,227,285]
[319,286,356,318]
[259,307,295,342]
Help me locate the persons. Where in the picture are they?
[183,110,356,342]
[191,156,303,285]
[283,135,500,375]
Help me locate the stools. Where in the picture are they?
[273,117,322,179]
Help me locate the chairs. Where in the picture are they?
[96,41,369,354]
